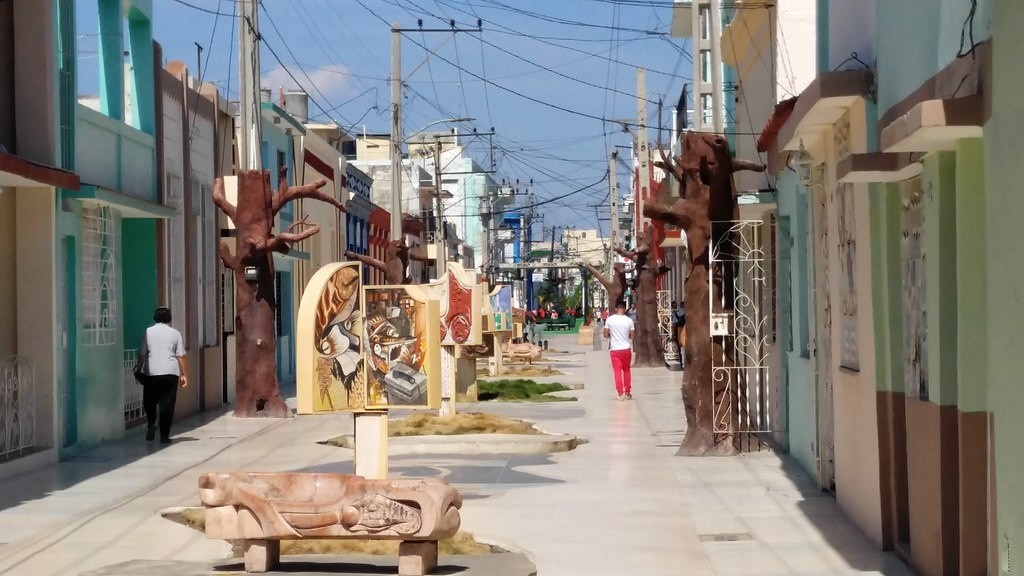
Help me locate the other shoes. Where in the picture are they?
[146,425,156,441]
[160,435,172,443]
[624,393,632,399]
[619,395,623,401]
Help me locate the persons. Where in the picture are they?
[603,300,635,401]
[531,301,685,368]
[137,306,188,444]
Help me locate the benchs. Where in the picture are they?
[546,323,569,331]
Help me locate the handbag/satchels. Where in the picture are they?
[133,365,143,384]
